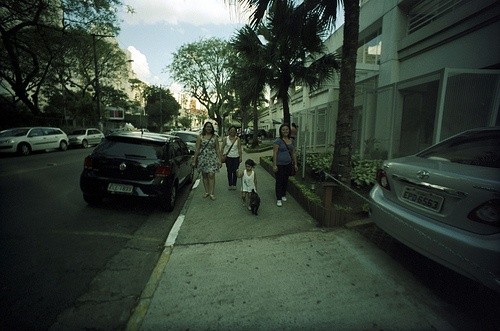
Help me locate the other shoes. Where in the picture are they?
[210,194,216,200]
[232,185,237,190]
[277,200,282,206]
[204,192,209,198]
[247,205,251,210]
[282,197,286,201]
[243,202,246,206]
[228,185,232,190]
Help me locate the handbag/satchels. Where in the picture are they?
[222,154,227,162]
[195,156,198,168]
[289,162,296,176]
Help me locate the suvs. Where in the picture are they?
[192,126,199,132]
[80,130,195,212]
[170,131,200,154]
[69,128,105,148]
[0,127,70,156]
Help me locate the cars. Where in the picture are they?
[368,121,500,292]
[136,128,149,132]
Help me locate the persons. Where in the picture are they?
[236,158,258,210]
[220,124,242,190]
[273,123,298,206]
[237,125,254,143]
[290,122,297,140]
[191,120,222,200]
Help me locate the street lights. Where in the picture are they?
[97,60,134,128]
